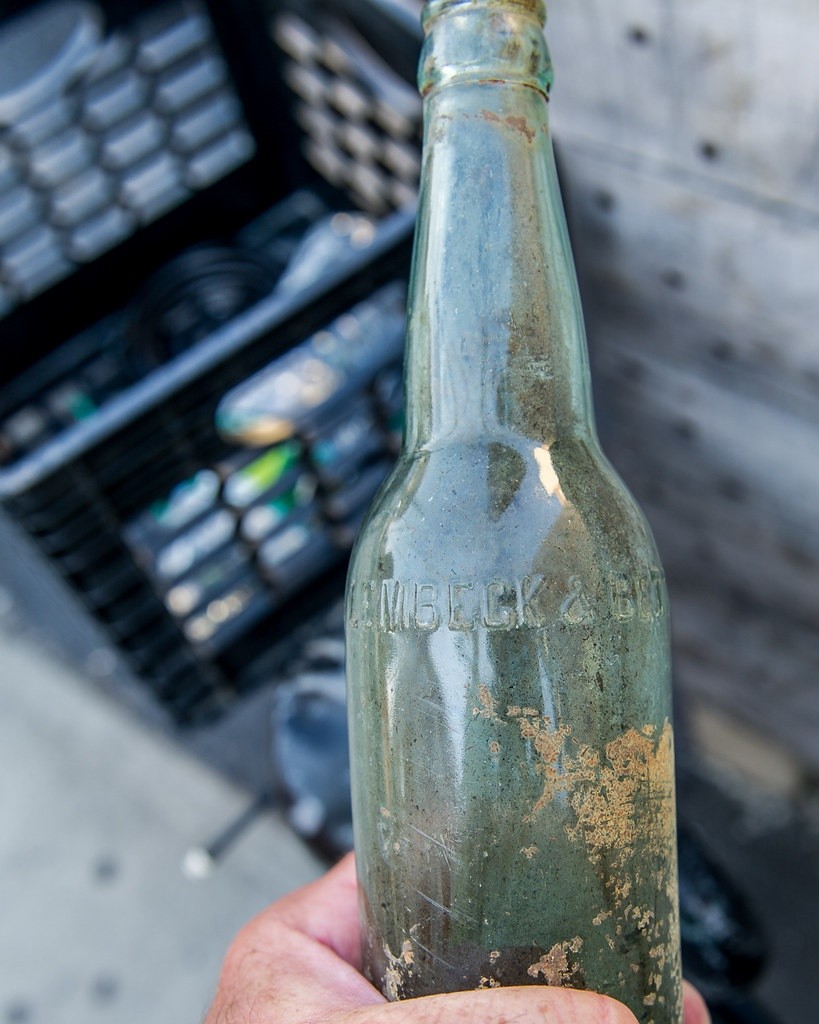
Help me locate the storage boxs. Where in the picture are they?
[0,2,579,731]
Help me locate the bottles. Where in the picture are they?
[344,0,682,1024]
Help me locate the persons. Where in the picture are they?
[201,841,713,1024]
